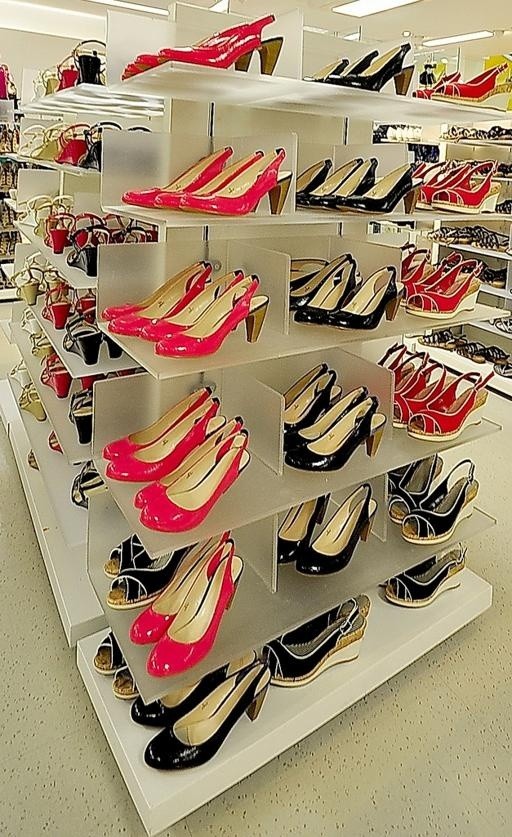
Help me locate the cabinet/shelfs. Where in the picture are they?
[401,110,512,401]
[4,11,493,833]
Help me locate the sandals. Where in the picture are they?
[488,319,512,334]
[479,345,510,363]
[494,361,512,379]
[455,342,485,363]
[496,199,512,214]
[427,225,512,256]
[473,160,512,178]
[483,267,507,289]
[453,335,467,347]
[418,330,456,350]
[439,126,512,144]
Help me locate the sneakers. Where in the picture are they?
[0,232,9,256]
[2,205,15,227]
[9,231,21,253]
[373,125,421,143]
[5,277,16,289]
[0,123,12,154]
[408,144,439,163]
[0,269,4,290]
[0,160,14,197]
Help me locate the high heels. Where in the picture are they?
[48,430,64,455]
[127,126,153,133]
[153,151,264,208]
[378,555,436,587]
[140,224,157,242]
[135,50,253,71]
[290,254,352,310]
[296,483,377,577]
[379,343,407,369]
[58,55,80,90]
[322,159,379,211]
[393,352,430,397]
[20,307,42,335]
[328,266,405,330]
[30,124,72,161]
[400,251,464,307]
[141,270,244,342]
[53,194,74,213]
[104,334,123,359]
[93,630,126,675]
[40,354,72,399]
[44,213,76,254]
[294,259,363,325]
[72,213,104,245]
[34,204,66,238]
[112,666,140,700]
[277,594,371,645]
[260,598,367,687]
[56,124,91,164]
[22,252,47,271]
[112,227,148,243]
[409,371,495,442]
[401,459,480,546]
[29,332,54,360]
[136,416,243,508]
[79,375,96,389]
[283,370,342,438]
[103,214,125,243]
[73,41,107,85]
[334,163,424,215]
[106,397,226,483]
[290,259,329,291]
[17,125,45,158]
[328,44,415,96]
[109,263,213,334]
[284,396,387,472]
[104,387,212,460]
[393,362,455,428]
[10,268,44,306]
[159,15,283,74]
[295,159,333,205]
[67,226,110,276]
[299,158,363,209]
[43,269,65,290]
[103,532,150,578]
[412,72,462,100]
[143,663,272,771]
[324,50,379,83]
[19,383,47,422]
[415,164,471,210]
[156,274,269,358]
[399,241,417,252]
[284,386,368,451]
[132,650,257,726]
[72,462,104,509]
[140,429,249,533]
[406,258,482,319]
[280,362,328,410]
[431,161,501,214]
[385,546,467,608]
[302,59,349,83]
[63,315,103,365]
[101,261,204,321]
[79,121,122,173]
[127,63,142,75]
[36,84,46,100]
[129,531,231,644]
[401,248,435,287]
[388,453,443,525]
[17,195,52,227]
[181,149,293,215]
[146,539,244,676]
[431,64,512,113]
[411,163,426,177]
[417,162,446,184]
[106,543,198,610]
[42,285,70,329]
[277,493,331,566]
[121,146,233,208]
[73,289,96,325]
[68,388,92,444]
[9,360,32,389]
[42,69,58,95]
[387,465,410,502]
